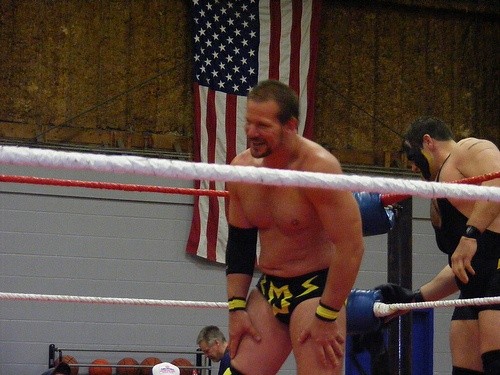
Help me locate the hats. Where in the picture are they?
[42,363,71,375]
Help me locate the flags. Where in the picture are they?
[186,0,320,270]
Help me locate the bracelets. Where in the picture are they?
[315,300,338,321]
[227,297,247,312]
[462,224,482,240]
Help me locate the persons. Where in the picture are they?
[222,81,364,375]
[196,325,232,375]
[383,116,500,375]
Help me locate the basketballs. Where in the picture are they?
[54,355,194,375]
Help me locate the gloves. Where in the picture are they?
[375,283,426,305]
